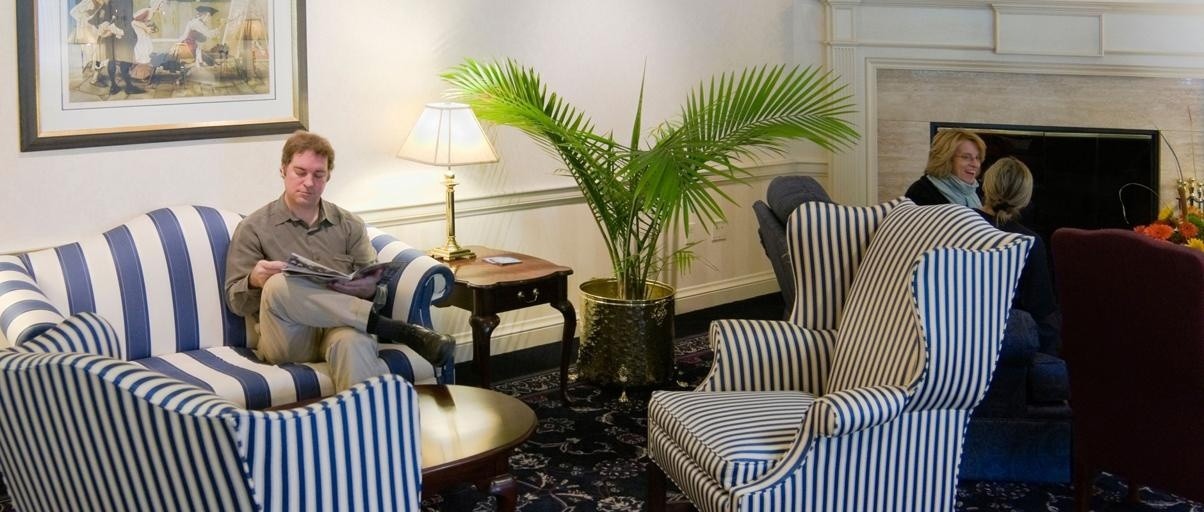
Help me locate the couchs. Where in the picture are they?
[752,174,1073,486]
[0,203,456,411]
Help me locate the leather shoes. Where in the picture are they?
[403,324,455,367]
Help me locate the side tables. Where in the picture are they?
[423,245,578,406]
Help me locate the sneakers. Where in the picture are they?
[125,85,144,94]
[109,83,118,92]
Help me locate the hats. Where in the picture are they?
[196,6,218,15]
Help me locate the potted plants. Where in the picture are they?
[437,52,864,397]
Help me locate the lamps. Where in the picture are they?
[397,100,501,261]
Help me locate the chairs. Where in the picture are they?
[1051,225,1204,512]
[645,198,1038,512]
[0,313,424,512]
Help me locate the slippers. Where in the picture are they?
[89,78,106,88]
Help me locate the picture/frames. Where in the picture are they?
[15,1,310,153]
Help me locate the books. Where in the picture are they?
[280,249,408,284]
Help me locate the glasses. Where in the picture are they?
[954,152,981,162]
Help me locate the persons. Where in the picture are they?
[223,129,456,396]
[67,0,220,96]
[970,155,1057,326]
[903,126,987,210]
